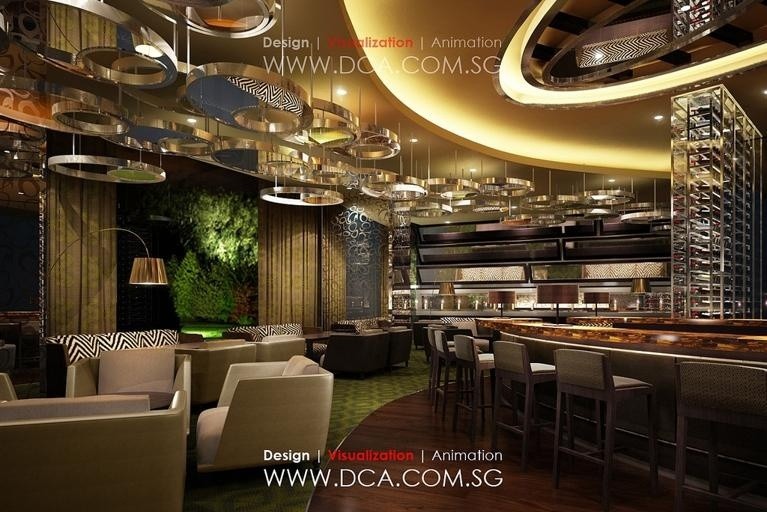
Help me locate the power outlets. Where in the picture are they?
[1,343,16,369]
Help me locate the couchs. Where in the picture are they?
[440,316,476,335]
[223,323,328,360]
[249,334,306,360]
[196,355,335,473]
[175,340,258,403]
[412,318,441,349]
[44,328,205,395]
[323,330,391,378]
[65,345,192,434]
[385,326,413,368]
[0,372,18,400]
[0,321,40,359]
[332,316,393,334]
[0,390,188,511]
[394,318,410,329]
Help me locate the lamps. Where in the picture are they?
[537,284,579,324]
[45,226,168,285]
[432,270,455,295]
[583,291,610,316]
[130,31,163,57]
[631,278,652,293]
[488,290,515,317]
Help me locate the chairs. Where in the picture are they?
[492,340,577,471]
[422,326,436,400]
[453,333,520,444]
[553,347,661,512]
[671,354,767,512]
[434,329,476,423]
